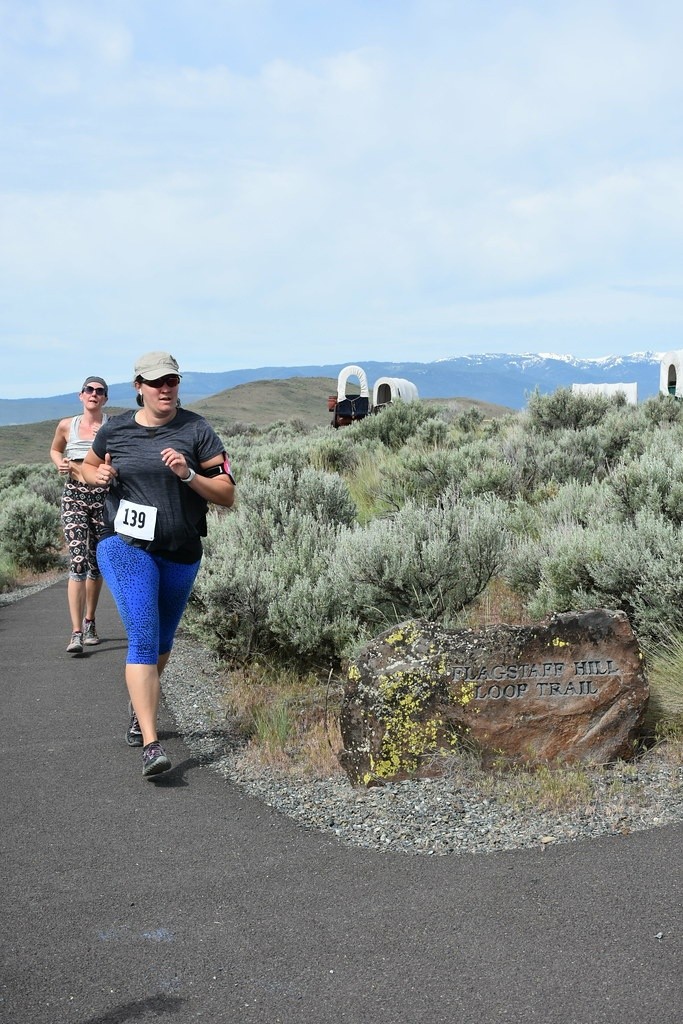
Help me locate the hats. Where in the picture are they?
[81,375,108,397]
[133,352,183,383]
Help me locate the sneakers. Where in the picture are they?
[81,618,99,645]
[137,741,172,776]
[125,700,143,747]
[66,632,83,653]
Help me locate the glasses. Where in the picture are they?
[133,375,180,388]
[82,386,108,396]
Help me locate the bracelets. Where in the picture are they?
[180,468,196,482]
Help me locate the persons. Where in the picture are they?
[80,352,237,774]
[50,377,112,653]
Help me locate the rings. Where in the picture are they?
[102,475,104,479]
